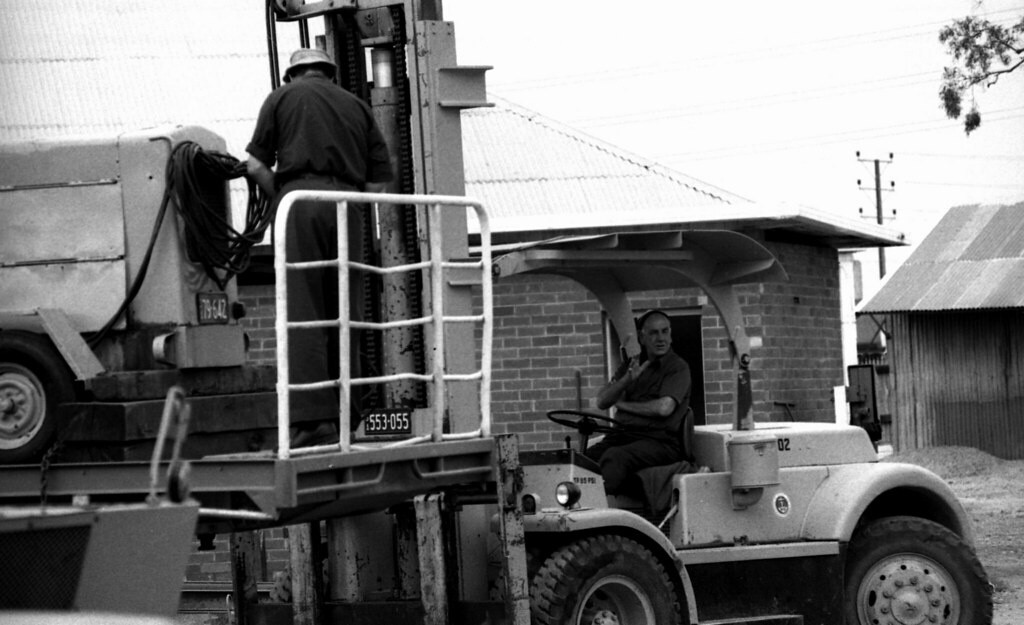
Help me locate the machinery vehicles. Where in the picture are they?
[264,0,996,624]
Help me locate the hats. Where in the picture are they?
[283,48,337,82]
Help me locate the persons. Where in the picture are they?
[580,310,691,494]
[246,49,392,452]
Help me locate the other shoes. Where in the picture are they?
[275,421,339,454]
